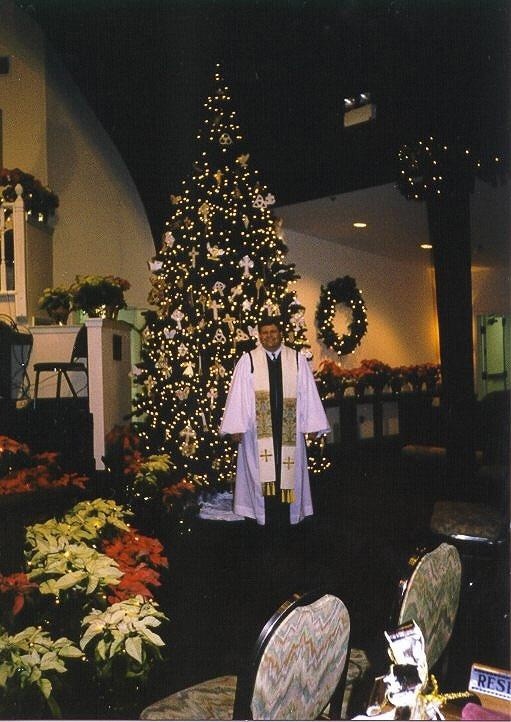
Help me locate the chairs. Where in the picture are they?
[32,325,92,411]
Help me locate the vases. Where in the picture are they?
[44,306,119,325]
[319,384,440,398]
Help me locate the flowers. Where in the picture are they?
[37,273,130,311]
[311,359,441,387]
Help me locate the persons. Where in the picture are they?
[218,316,332,527]
